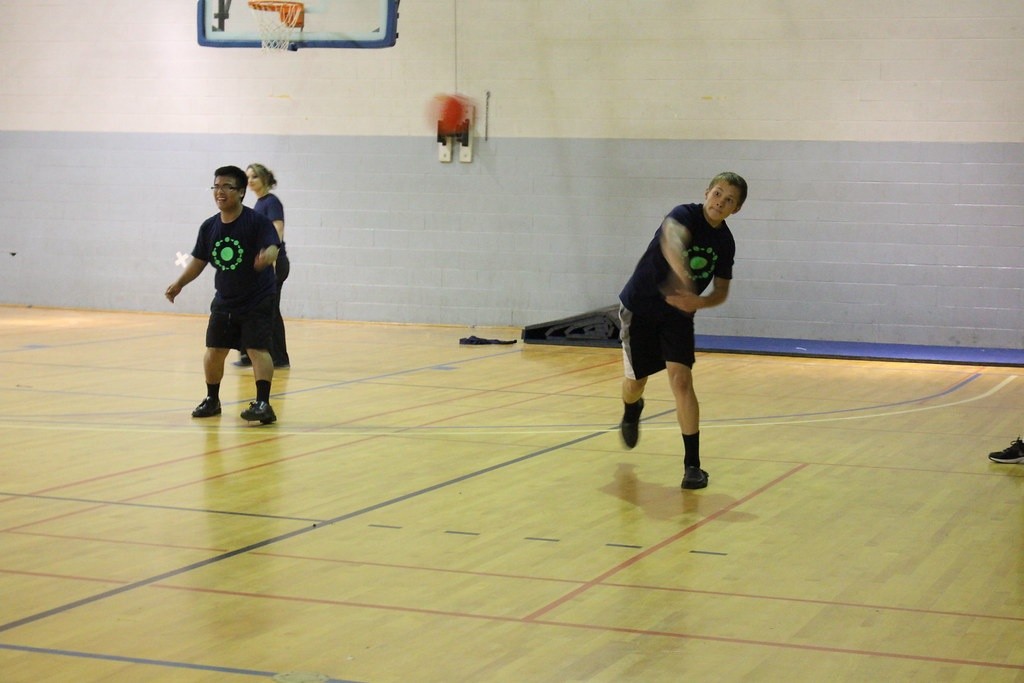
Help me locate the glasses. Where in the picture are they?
[211,184,243,193]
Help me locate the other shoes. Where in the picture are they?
[231,356,253,367]
[271,358,290,370]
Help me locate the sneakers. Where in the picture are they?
[191,395,222,417]
[680,463,709,490]
[621,397,645,449]
[240,399,277,424]
[987,435,1024,463]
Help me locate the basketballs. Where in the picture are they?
[427,95,462,131]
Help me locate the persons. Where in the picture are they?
[232,163,290,367]
[617,171,747,489]
[988,436,1024,465]
[164,166,280,424]
